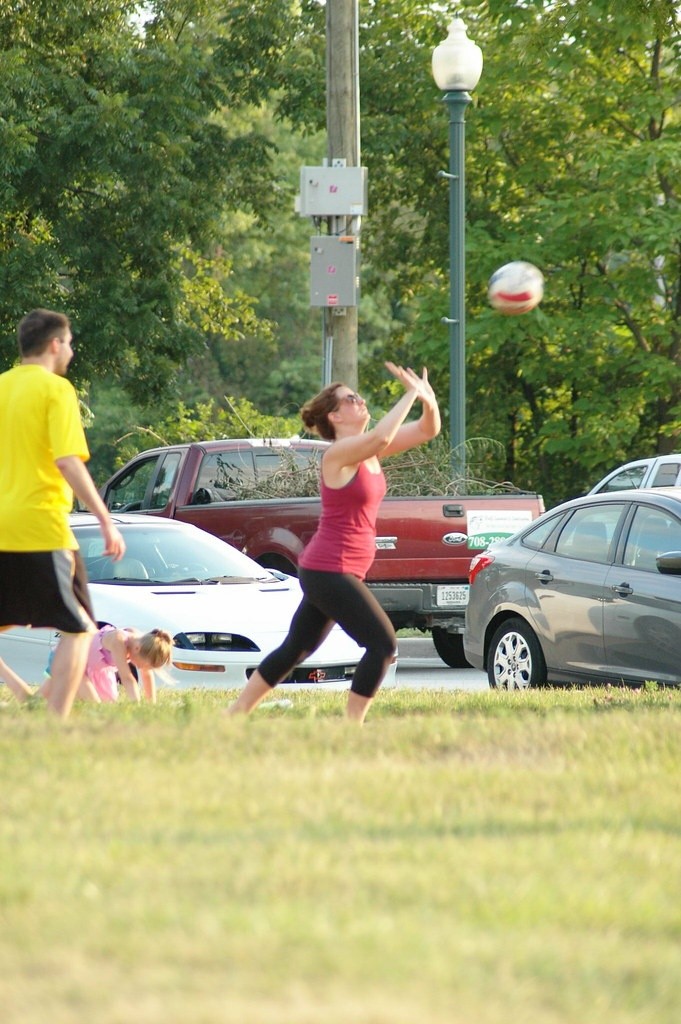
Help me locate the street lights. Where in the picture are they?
[433,10,485,494]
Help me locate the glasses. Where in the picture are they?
[333,392,360,414]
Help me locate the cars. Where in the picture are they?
[463,452,681,691]
[0,513,397,706]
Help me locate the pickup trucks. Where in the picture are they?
[103,437,546,666]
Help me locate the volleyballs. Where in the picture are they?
[487,260,545,317]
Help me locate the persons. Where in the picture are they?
[225,362,441,721]
[0,309,170,717]
